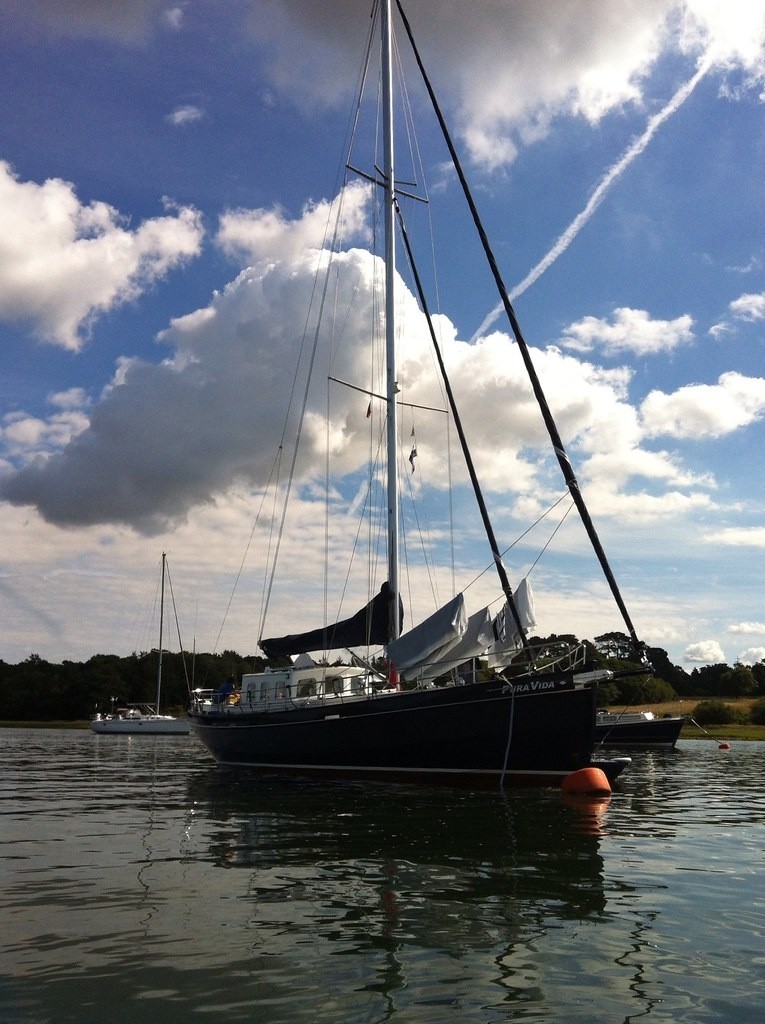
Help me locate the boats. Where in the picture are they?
[592,710,687,749]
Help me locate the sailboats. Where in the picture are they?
[189,1,637,793]
[90,553,194,737]
